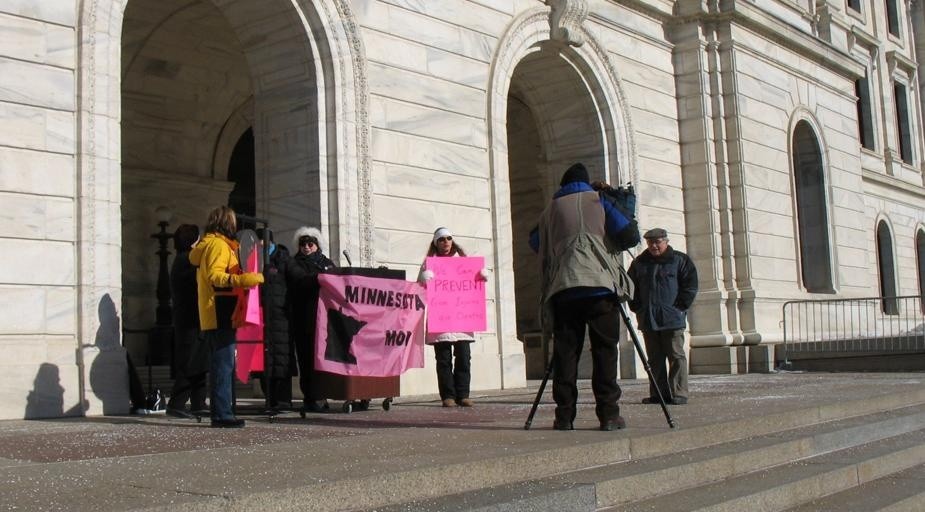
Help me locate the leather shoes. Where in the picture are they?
[642,395,668,404]
[443,398,455,406]
[212,418,246,428]
[191,406,211,417]
[166,407,193,419]
[600,416,625,431]
[553,419,573,429]
[458,398,474,406]
[672,395,687,405]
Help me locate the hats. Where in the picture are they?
[643,228,667,238]
[173,223,199,251]
[560,164,590,186]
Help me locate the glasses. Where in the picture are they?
[438,236,452,242]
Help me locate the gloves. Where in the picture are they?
[420,271,433,283]
[480,268,491,281]
[233,272,264,288]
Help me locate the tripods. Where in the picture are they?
[525,298,675,430]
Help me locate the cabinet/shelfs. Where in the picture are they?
[305,266,407,410]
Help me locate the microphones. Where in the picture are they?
[342,249,352,266]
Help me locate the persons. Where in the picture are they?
[626,228,698,404]
[417,227,493,407]
[528,162,642,431]
[254,228,314,409]
[292,227,336,412]
[165,223,211,418]
[189,206,264,428]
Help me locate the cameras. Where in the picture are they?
[593,180,635,216]
